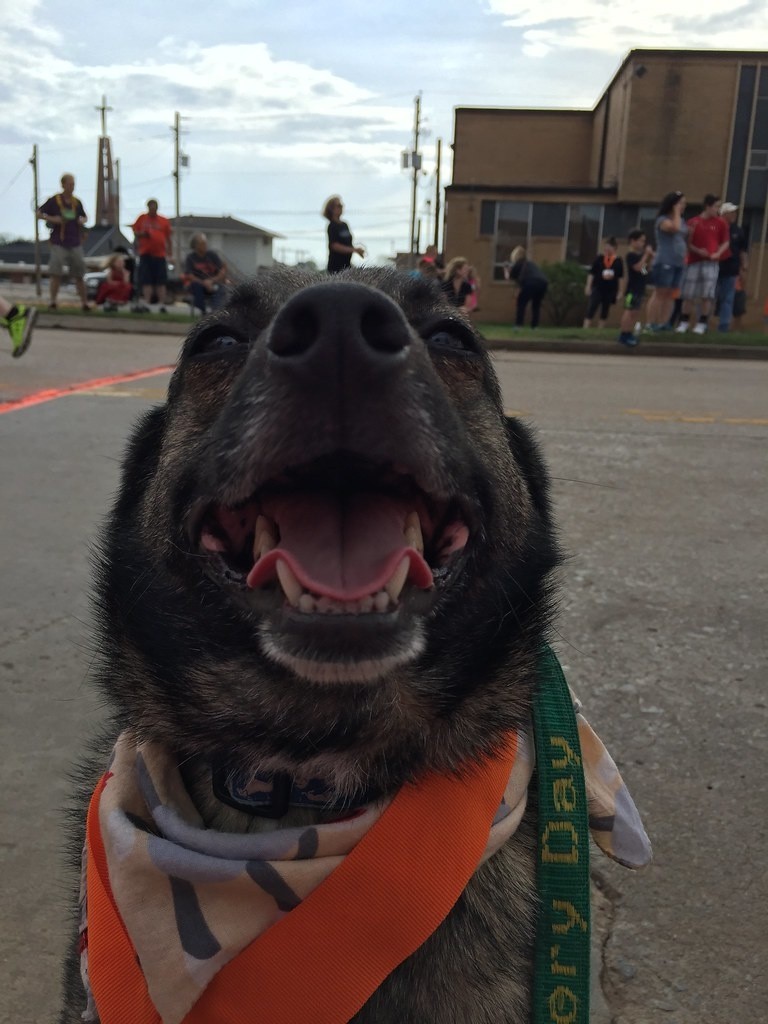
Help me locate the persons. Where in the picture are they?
[583,236,624,329]
[509,246,549,333]
[441,256,480,311]
[322,195,365,275]
[673,194,732,335]
[0,298,38,360]
[695,202,749,334]
[419,245,447,281]
[37,173,93,314]
[185,233,228,317]
[613,230,656,347]
[131,198,172,315]
[646,191,688,332]
[96,255,132,305]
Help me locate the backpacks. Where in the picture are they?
[55,195,81,248]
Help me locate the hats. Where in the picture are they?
[719,203,739,215]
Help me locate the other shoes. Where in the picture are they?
[82,304,92,312]
[159,306,167,313]
[143,307,151,313]
[616,331,638,346]
[49,304,58,311]
[693,323,707,335]
[675,321,689,333]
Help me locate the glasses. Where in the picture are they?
[676,191,681,197]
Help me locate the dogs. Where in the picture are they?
[62,269,621,1024]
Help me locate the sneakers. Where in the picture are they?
[0,304,39,359]
